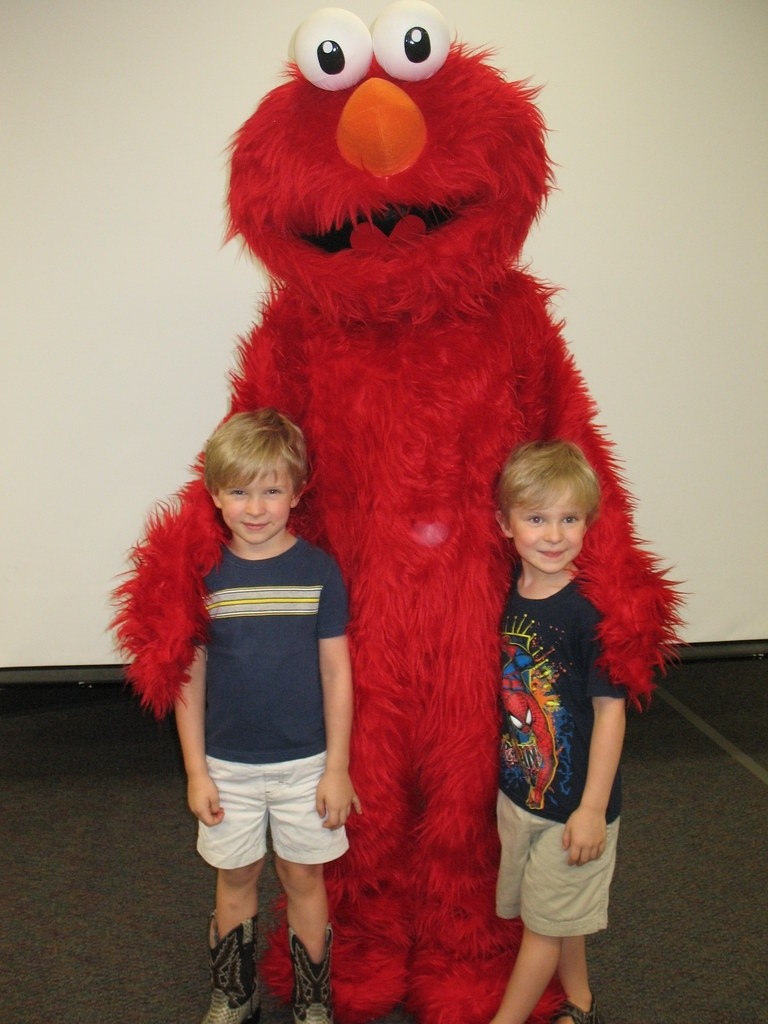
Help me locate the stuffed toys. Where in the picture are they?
[108,0,680,1024]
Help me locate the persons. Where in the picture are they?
[174,411,362,1024]
[489,437,629,1024]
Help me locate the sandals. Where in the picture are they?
[552,994,605,1024]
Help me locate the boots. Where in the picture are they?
[288,922,333,1024]
[200,910,260,1024]
